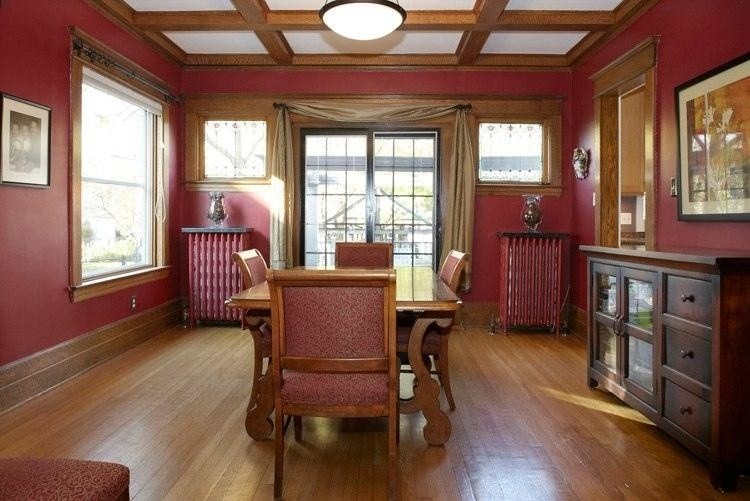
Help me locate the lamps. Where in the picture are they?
[318,0,408,44]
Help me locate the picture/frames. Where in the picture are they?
[672,51,750,223]
[0,92,54,193]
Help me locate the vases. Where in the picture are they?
[519,191,543,229]
[205,190,229,226]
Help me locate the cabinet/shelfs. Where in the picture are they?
[621,91,647,198]
[579,242,750,495]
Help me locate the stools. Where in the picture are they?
[1,454,130,500]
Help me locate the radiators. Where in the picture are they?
[495,231,569,337]
[181,226,253,331]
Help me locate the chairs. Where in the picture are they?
[232,248,474,410]
[265,270,407,501]
[334,242,394,271]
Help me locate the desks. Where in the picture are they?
[224,266,463,448]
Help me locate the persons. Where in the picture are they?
[10,119,42,174]
